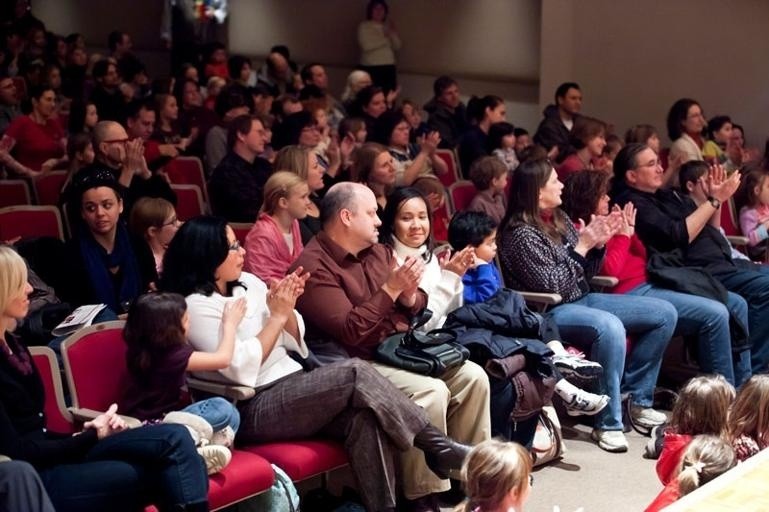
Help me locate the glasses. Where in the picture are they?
[229,240,241,252]
[160,218,179,228]
[633,158,662,168]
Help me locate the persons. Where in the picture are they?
[1,1,768,512]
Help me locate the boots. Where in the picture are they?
[163,412,213,448]
[415,424,477,481]
[196,427,234,476]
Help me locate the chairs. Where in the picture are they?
[187,377,349,512]
[28,345,159,512]
[61,321,276,512]
[0,156,257,273]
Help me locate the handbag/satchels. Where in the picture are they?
[529,398,566,469]
[375,308,470,376]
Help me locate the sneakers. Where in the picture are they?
[550,351,605,384]
[566,389,612,417]
[644,424,666,459]
[591,429,629,453]
[631,404,667,427]
